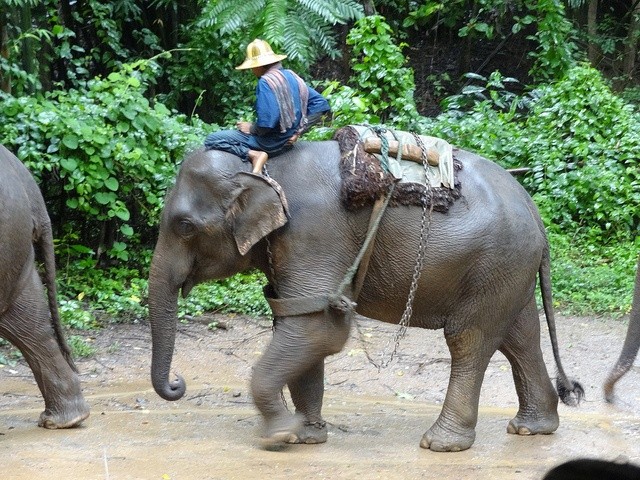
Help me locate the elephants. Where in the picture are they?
[0,143,90,428]
[148,140,583,452]
[603,259,640,400]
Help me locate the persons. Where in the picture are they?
[202,37,332,175]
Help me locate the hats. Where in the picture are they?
[235,39,287,70]
